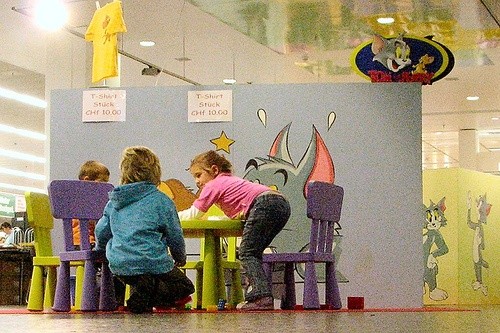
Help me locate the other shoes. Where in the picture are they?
[126,292,152,314]
[235,294,274,310]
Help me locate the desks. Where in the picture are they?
[124,219,245,308]
[0,246,31,305]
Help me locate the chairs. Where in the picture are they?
[262,180,344,309]
[49,179,116,313]
[178,236,243,309]
[25,191,86,311]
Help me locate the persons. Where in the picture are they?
[73,159,126,309]
[20,240,37,247]
[176,149,291,311]
[1,221,18,248]
[94,145,195,313]
